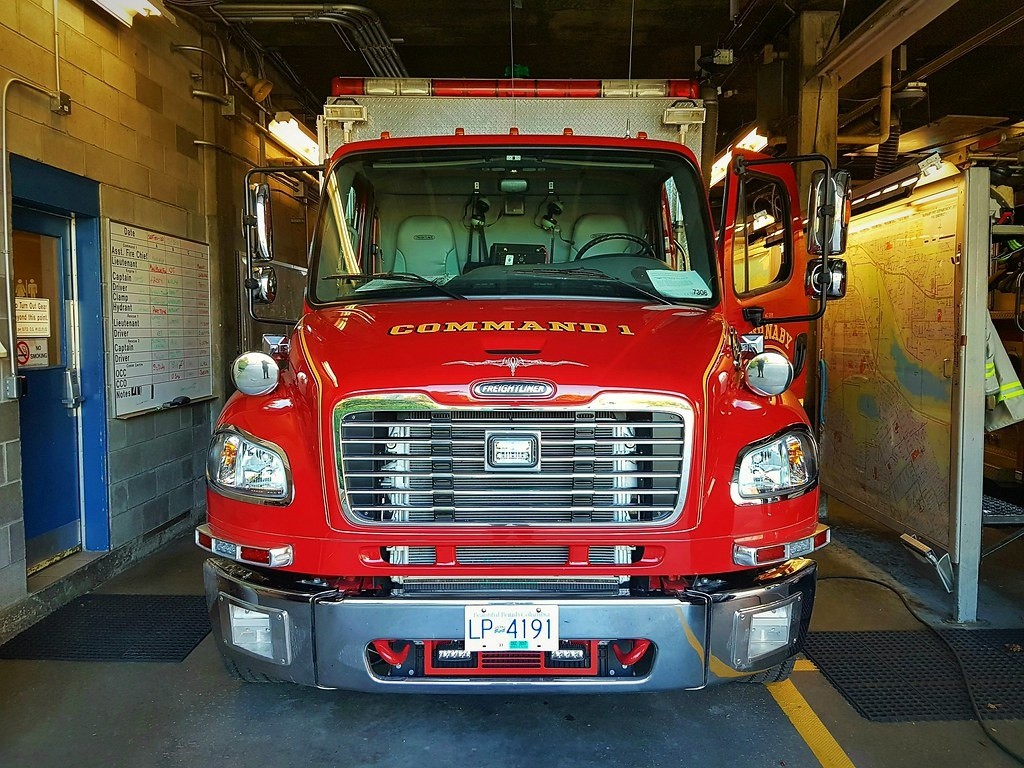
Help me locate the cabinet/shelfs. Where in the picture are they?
[982,225,1024,486]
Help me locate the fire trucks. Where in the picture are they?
[195,76,852,696]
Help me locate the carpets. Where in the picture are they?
[802,628,1024,723]
[0,592,212,665]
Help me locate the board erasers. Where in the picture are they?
[163,396,191,408]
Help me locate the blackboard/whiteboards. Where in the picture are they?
[101,216,215,420]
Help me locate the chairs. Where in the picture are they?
[568,214,632,262]
[393,214,461,277]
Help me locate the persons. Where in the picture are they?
[757,358,765,378]
[261,360,271,379]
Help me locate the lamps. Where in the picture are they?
[710,118,776,189]
[91,0,179,30]
